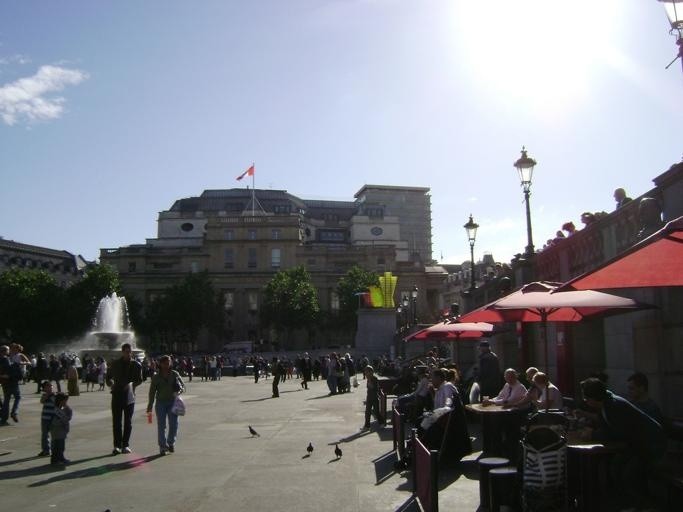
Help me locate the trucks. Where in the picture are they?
[222,341,255,353]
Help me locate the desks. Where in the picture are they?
[464,403,519,450]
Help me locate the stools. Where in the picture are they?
[478,457,510,508]
[489,467,518,512]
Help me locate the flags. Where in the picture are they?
[235,165,254,181]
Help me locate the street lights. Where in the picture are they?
[463,212,481,290]
[395,303,403,329]
[509,143,540,255]
[409,283,420,326]
[401,294,409,327]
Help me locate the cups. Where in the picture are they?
[146,412,152,423]
[483,396,489,406]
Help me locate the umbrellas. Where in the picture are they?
[452,277,660,416]
[402,321,495,343]
[546,213,682,295]
[426,314,502,334]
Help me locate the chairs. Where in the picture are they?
[520,426,572,512]
[420,394,461,464]
[401,386,435,423]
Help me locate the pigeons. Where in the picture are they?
[335,444,342,459]
[307,442,313,453]
[248,426,260,438]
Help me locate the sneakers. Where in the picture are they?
[112,448,121,454]
[160,449,166,456]
[122,447,130,453]
[169,444,175,452]
[38,452,49,457]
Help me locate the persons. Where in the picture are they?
[132,352,194,381]
[106,344,144,454]
[579,377,666,510]
[38,381,73,465]
[271,350,391,398]
[22,351,114,395]
[3,342,31,426]
[626,372,663,419]
[362,365,385,431]
[395,347,465,451]
[147,354,182,456]
[200,351,270,382]
[483,365,562,447]
